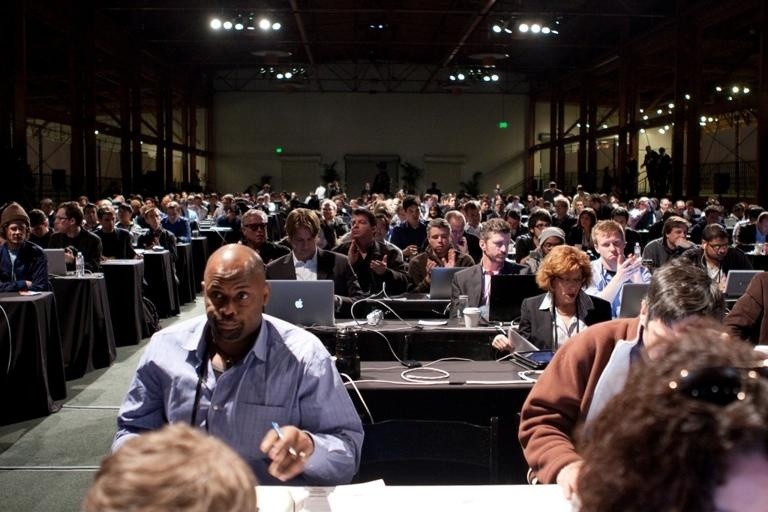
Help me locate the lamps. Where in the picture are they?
[492,15,559,36]
[259,66,307,81]
[450,64,499,82]
[209,12,281,33]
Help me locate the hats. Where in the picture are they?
[0,201,30,227]
[538,226,565,249]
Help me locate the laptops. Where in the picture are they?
[721,269,764,297]
[264,279,335,329]
[483,274,547,324]
[42,248,74,275]
[426,267,465,300]
[619,282,650,318]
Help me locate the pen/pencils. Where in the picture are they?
[271,421,298,458]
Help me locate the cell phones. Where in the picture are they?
[401,359,421,367]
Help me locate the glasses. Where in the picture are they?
[244,223,267,231]
[667,367,768,407]
[709,244,728,249]
[556,276,584,283]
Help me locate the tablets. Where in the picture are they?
[514,348,562,368]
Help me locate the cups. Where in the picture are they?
[462,307,481,328]
[192,230,199,238]
[207,215,211,219]
[508,244,514,252]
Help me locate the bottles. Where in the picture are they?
[75,251,84,277]
[457,295,469,325]
[634,242,641,258]
[366,309,383,325]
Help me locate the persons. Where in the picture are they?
[301,187,767,352]
[518,264,726,500]
[658,148,672,195]
[111,243,364,486]
[623,154,638,196]
[640,146,659,195]
[83,421,257,511]
[1,186,301,292]
[577,317,768,512]
